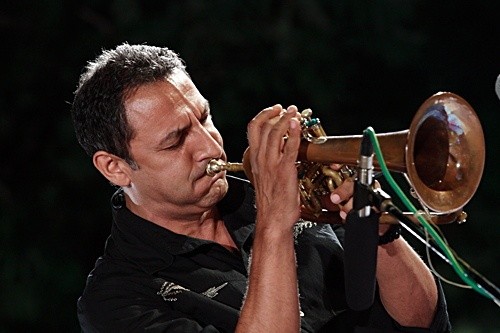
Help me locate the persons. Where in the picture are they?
[69,43,452,333]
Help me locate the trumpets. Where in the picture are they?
[206,91,485,224]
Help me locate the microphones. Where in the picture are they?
[343,130,380,309]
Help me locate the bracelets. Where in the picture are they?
[377,222,404,246]
